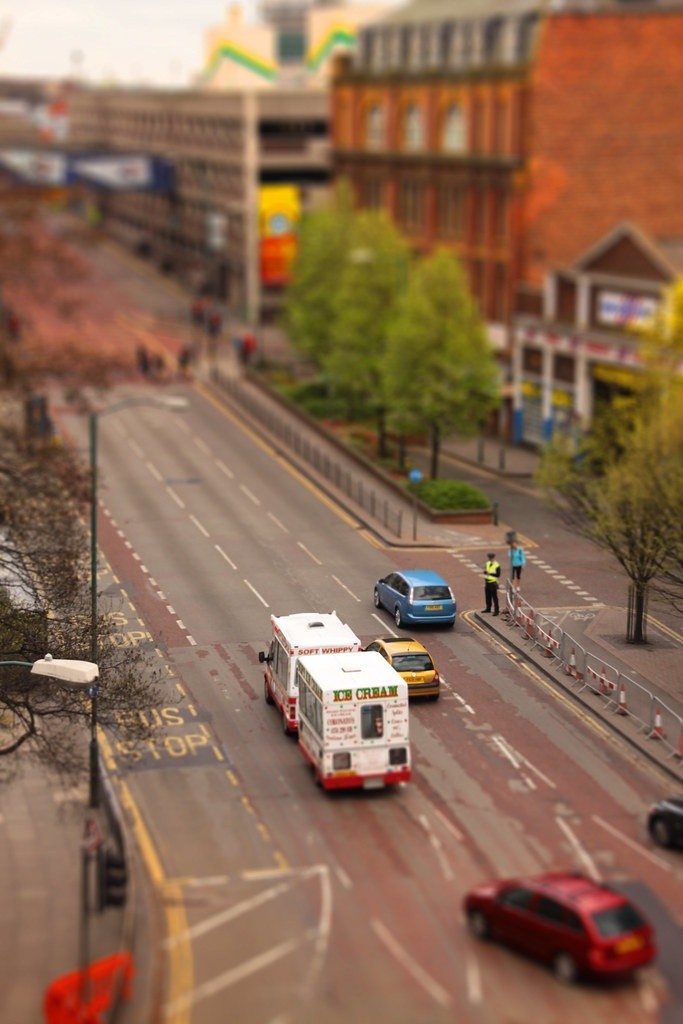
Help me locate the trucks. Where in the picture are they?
[297,650,412,794]
[257,611,361,735]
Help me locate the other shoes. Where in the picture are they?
[493,611,500,616]
[481,608,491,613]
[516,586,520,592]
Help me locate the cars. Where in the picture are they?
[374,569,456,630]
[648,796,682,853]
[361,636,439,701]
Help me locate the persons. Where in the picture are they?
[481,552,500,616]
[507,542,525,592]
[136,346,190,379]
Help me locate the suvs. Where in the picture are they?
[462,871,658,985]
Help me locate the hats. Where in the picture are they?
[487,553,495,558]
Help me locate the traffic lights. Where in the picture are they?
[98,848,128,914]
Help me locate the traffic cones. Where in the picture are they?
[649,707,667,740]
[540,629,556,657]
[502,584,525,626]
[564,648,579,676]
[592,667,611,696]
[614,683,630,716]
[520,609,538,640]
[672,725,683,760]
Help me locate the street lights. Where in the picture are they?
[3,389,193,1000]
